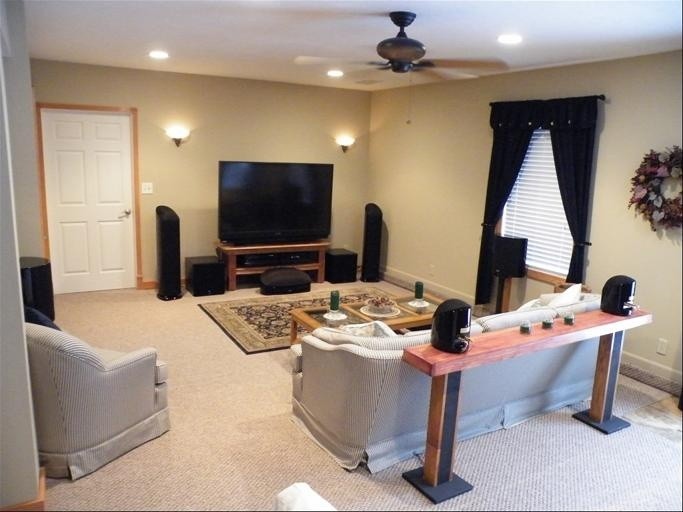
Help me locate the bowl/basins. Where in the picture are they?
[365,298,398,312]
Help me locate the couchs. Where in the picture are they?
[291,281,604,474]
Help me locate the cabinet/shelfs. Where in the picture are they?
[215,240,329,291]
[18,255,53,320]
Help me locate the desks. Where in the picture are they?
[396,306,657,506]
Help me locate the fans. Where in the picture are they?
[292,11,510,81]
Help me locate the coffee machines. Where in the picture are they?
[600,275,640,316]
[429,299,473,355]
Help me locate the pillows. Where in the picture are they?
[546,285,581,306]
[25,305,59,330]
[338,317,398,340]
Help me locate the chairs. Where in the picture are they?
[23,322,169,480]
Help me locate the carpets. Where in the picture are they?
[195,285,412,356]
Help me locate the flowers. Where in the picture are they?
[629,144,682,229]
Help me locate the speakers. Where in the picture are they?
[327,248,357,284]
[156,205,180,300]
[355,203,383,282]
[186,257,227,296]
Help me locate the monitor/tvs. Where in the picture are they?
[217,159,334,245]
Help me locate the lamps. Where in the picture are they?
[167,127,189,146]
[335,137,356,152]
